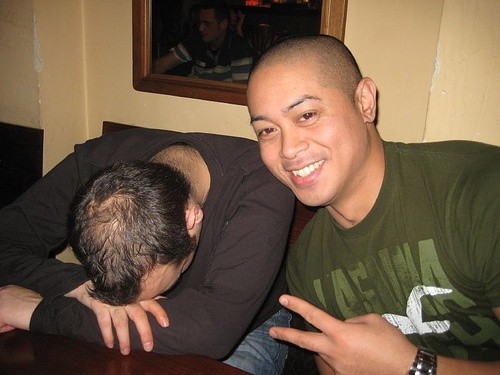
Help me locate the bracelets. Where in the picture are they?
[406,345,438,375]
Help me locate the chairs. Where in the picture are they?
[102,121,324,375]
[0,122,55,259]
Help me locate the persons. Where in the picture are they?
[154,3,252,87]
[0,130,299,375]
[247,34,500,375]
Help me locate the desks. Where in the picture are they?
[0,328,255,375]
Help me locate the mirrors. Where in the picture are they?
[132,0,348,106]
